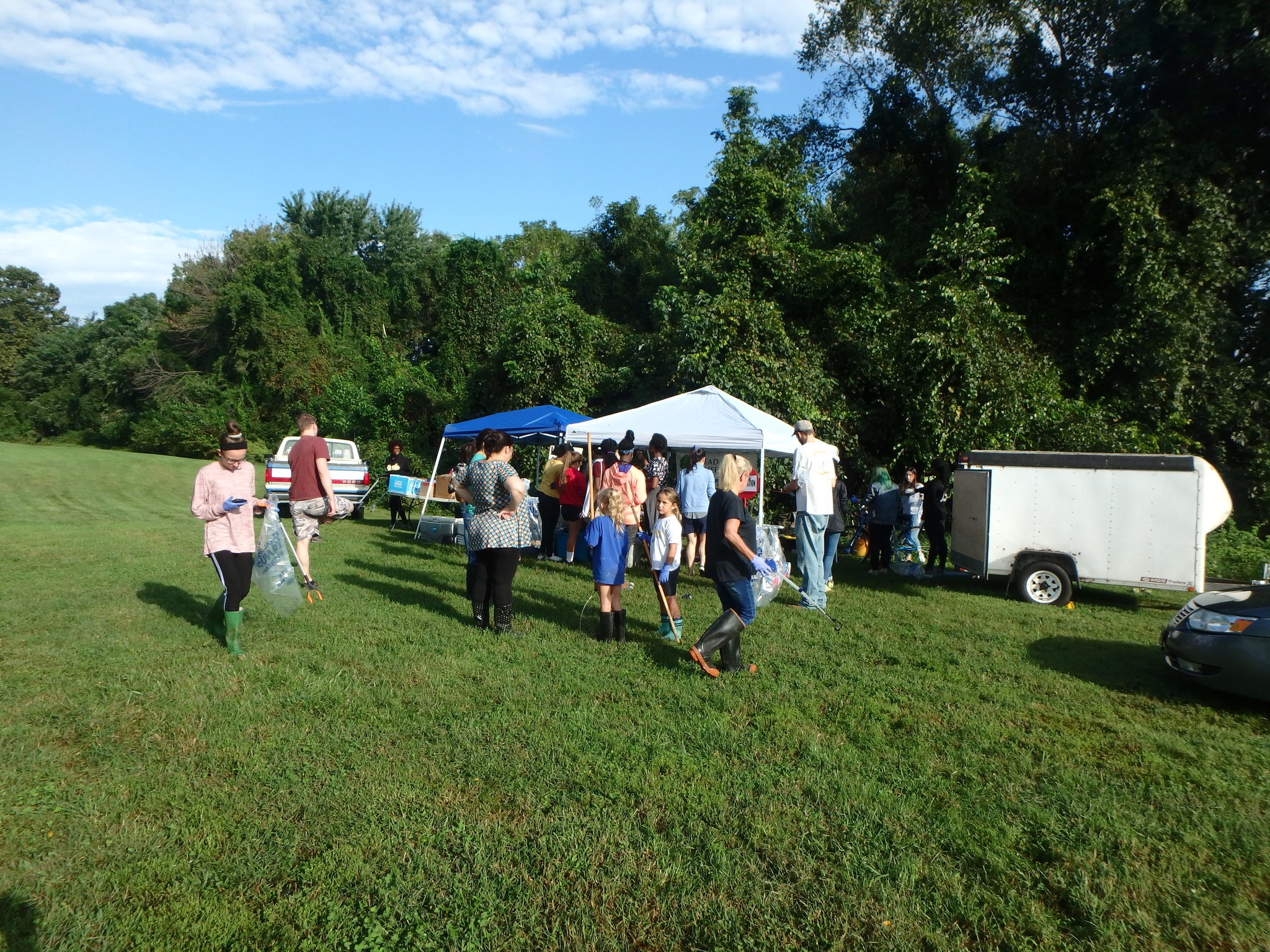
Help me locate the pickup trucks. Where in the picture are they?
[263,436,371,520]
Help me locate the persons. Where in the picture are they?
[385,439,412,528]
[637,487,684,640]
[190,421,280,658]
[850,457,950,579]
[535,430,716,590]
[451,428,531,636]
[689,454,772,677]
[784,420,847,608]
[288,413,354,589]
[584,488,629,643]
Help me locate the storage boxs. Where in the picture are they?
[435,474,455,500]
[419,482,436,497]
[457,518,465,546]
[388,474,417,495]
[414,478,425,495]
[420,515,458,545]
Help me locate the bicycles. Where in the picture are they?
[833,504,940,569]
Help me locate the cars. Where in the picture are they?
[1158,583,1270,704]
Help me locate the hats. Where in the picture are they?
[791,420,813,436]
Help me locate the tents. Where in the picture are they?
[537,385,838,563]
[414,405,595,539]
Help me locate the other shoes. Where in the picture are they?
[626,586,634,589]
[787,552,950,609]
[627,582,634,587]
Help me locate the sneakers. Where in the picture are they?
[699,565,704,577]
[537,553,546,559]
[303,581,319,589]
[311,534,322,543]
[694,556,701,564]
[546,553,562,562]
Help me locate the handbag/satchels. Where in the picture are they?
[525,494,542,548]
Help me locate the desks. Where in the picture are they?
[389,492,460,539]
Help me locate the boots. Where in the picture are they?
[224,607,247,657]
[471,601,492,630]
[612,609,626,643]
[593,609,613,643]
[206,589,227,646]
[493,603,525,638]
[661,607,758,676]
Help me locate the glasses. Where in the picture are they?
[222,453,247,463]
[746,474,751,480]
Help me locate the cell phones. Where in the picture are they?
[231,498,247,503]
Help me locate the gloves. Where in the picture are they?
[904,487,916,496]
[750,555,772,577]
[637,531,651,543]
[223,496,247,511]
[659,562,672,583]
